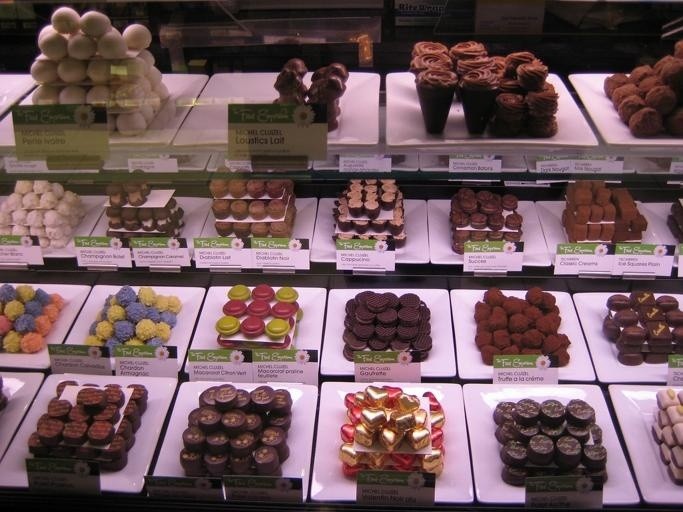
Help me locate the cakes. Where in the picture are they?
[342,290,433,361]
[492,397,608,487]
[603,288,682,367]
[409,38,561,140]
[271,56,348,133]
[177,383,293,483]
[331,179,409,249]
[650,388,683,486]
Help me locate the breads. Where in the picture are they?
[602,38,683,139]
[559,181,648,250]
[30,7,170,136]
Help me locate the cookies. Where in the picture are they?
[1,284,66,351]
[102,181,185,245]
[2,179,84,247]
[27,380,149,473]
[86,286,183,356]
[216,285,303,354]
[667,198,683,243]
[0,378,13,418]
[209,177,297,240]
[473,286,572,366]
[337,384,446,483]
[448,188,524,257]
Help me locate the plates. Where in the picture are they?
[0,74,209,144]
[193,198,317,260]
[63,284,207,371]
[572,293,683,383]
[642,203,683,246]
[0,373,178,495]
[310,382,474,503]
[568,73,683,146]
[426,200,551,265]
[320,288,457,377]
[609,385,683,506]
[0,195,110,257]
[311,198,430,262]
[184,287,327,372]
[535,200,659,266]
[90,197,214,260]
[0,283,92,369]
[450,289,596,381]
[172,72,380,147]
[0,372,45,464]
[463,384,640,503]
[153,381,317,501]
[386,72,599,145]
[1,73,37,119]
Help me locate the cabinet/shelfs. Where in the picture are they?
[1,74,682,510]
